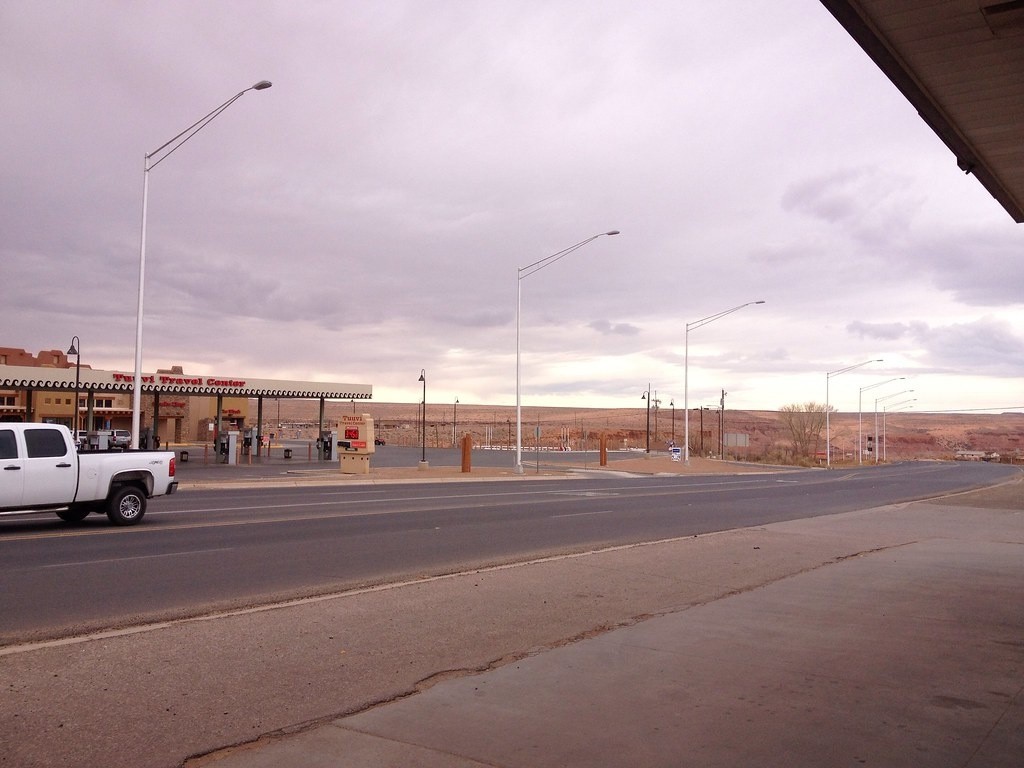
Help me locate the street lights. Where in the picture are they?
[883,397,917,461]
[452,395,461,448]
[692,406,710,451]
[669,398,677,443]
[825,358,884,466]
[418,368,427,462]
[128,76,274,450]
[67,335,79,441]
[513,231,623,476]
[874,389,915,463]
[857,377,906,468]
[640,391,651,454]
[683,300,766,461]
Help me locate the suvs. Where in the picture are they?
[375,436,386,445]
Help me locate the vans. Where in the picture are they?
[76,430,90,443]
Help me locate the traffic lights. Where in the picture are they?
[868,447,872,451]
[868,436,872,441]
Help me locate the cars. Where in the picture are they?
[979,454,990,461]
[74,441,81,451]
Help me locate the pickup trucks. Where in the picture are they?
[0,424,179,526]
[106,429,132,451]
